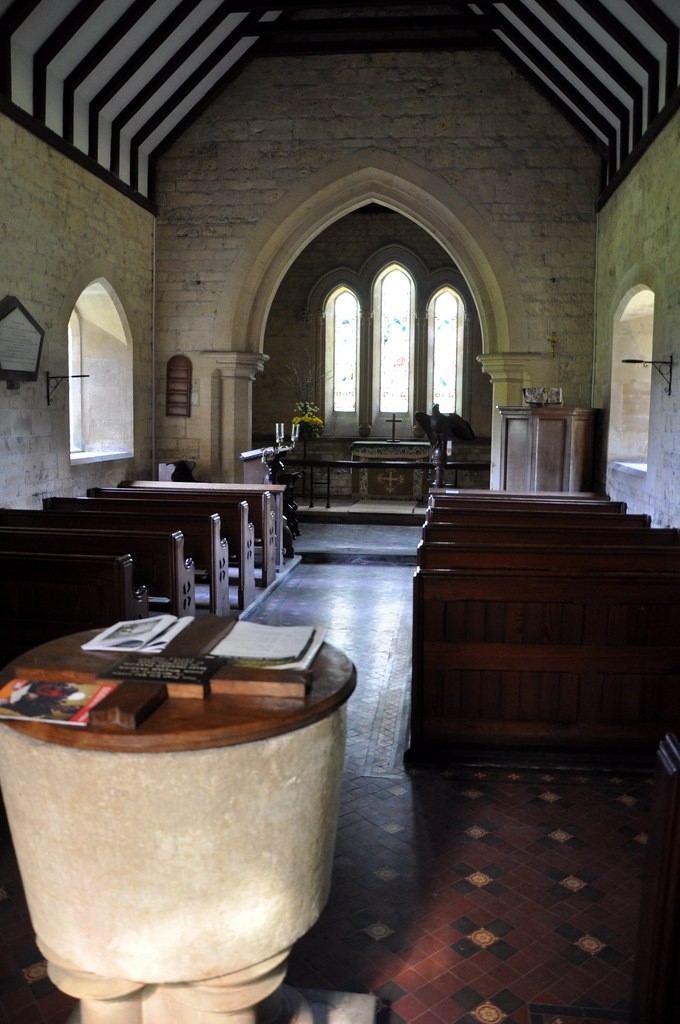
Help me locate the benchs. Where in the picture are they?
[0,479,288,664]
[401,493,680,775]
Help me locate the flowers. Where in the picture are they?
[291,400,326,438]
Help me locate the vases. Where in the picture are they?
[300,425,324,441]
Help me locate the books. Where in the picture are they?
[0,679,118,727]
[14,615,327,698]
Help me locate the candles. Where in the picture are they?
[276,423,300,438]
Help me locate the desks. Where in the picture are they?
[0,628,379,1024]
[349,440,434,505]
